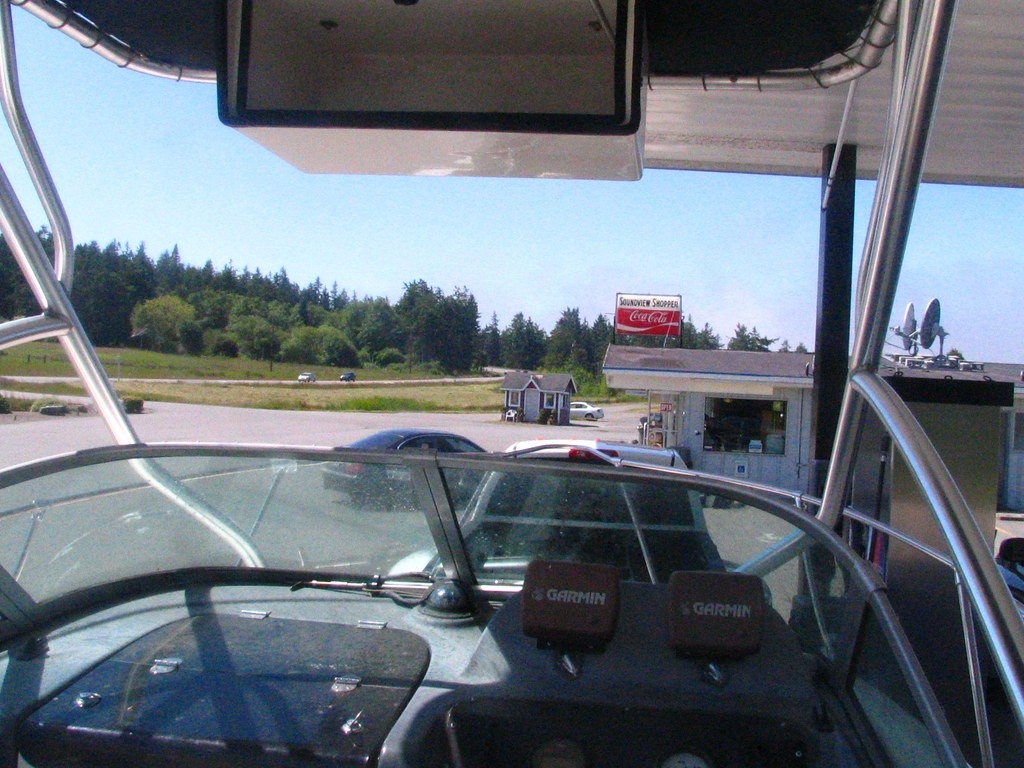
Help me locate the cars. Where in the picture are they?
[321,426,515,513]
[298,373,316,382]
[386,438,706,602]
[569,402,604,419]
[340,373,356,382]
